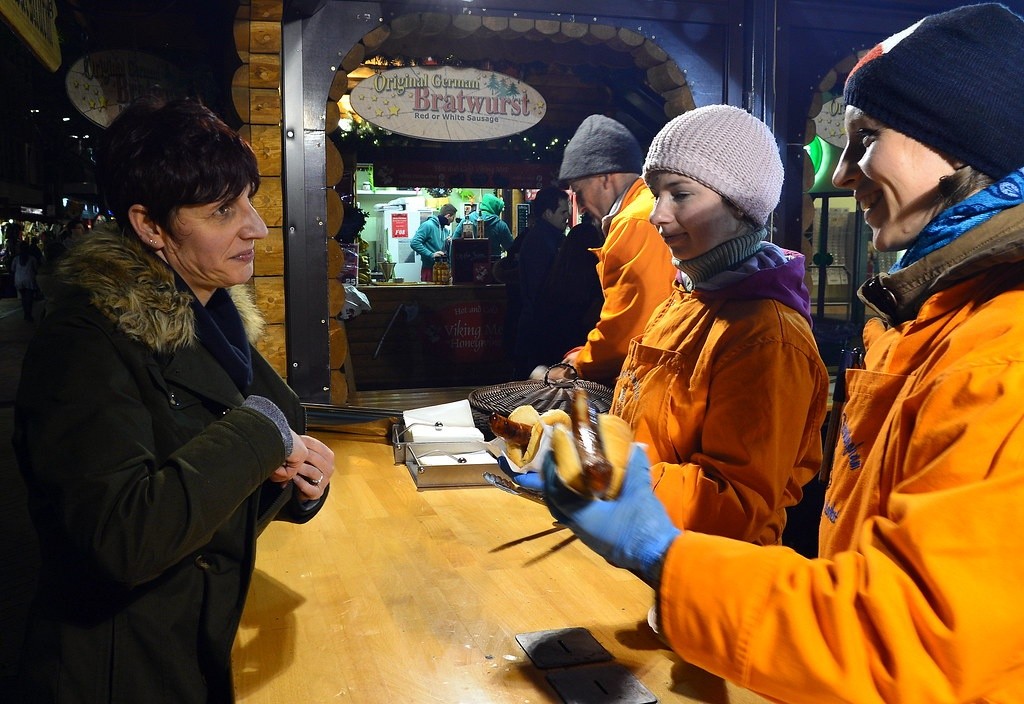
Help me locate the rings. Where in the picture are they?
[312,474,326,484]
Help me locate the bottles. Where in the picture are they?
[476,210,485,238]
[433,254,450,285]
[463,215,474,239]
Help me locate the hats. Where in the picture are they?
[844,4,1024,179]
[642,105,786,227]
[558,113,642,183]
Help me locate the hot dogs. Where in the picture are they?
[553,387,632,500]
[488,404,572,468]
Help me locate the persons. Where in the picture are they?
[506,189,579,379]
[865,249,882,287]
[410,203,457,282]
[564,206,604,340]
[542,0,1024,704]
[494,103,831,588]
[448,194,515,285]
[1,94,336,704]
[526,112,684,399]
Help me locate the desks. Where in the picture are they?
[344,281,509,390]
[230,387,788,704]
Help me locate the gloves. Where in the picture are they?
[544,444,681,583]
[495,459,544,492]
